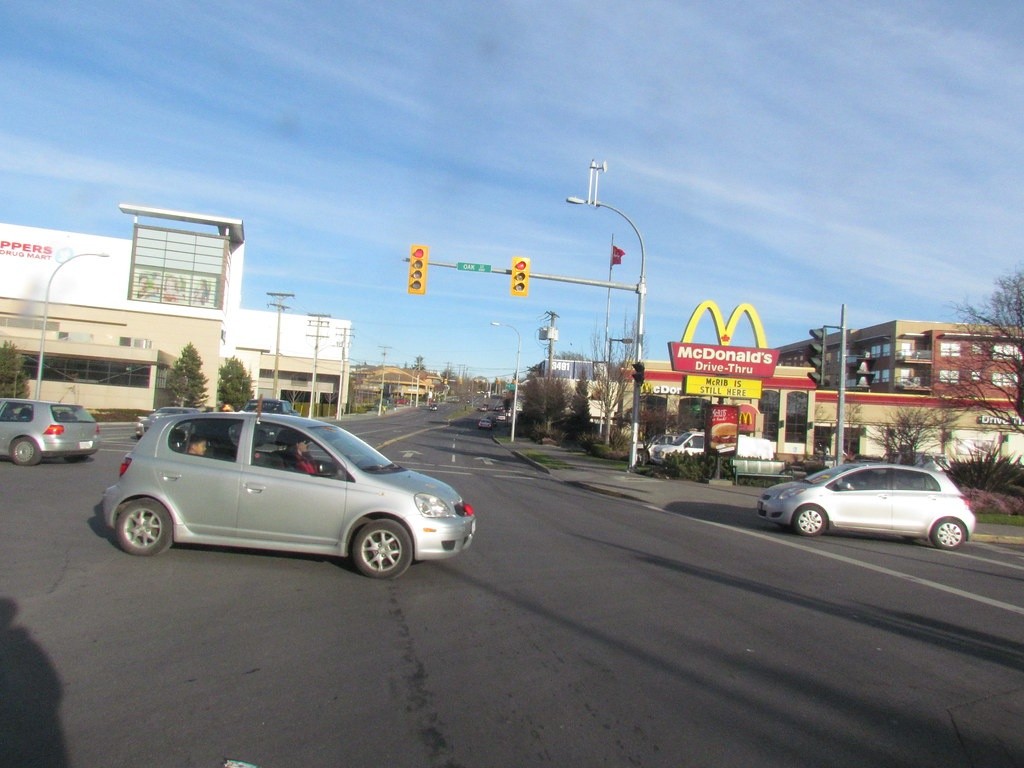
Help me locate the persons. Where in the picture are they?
[383,406,386,415]
[137,272,209,306]
[284,430,318,474]
[182,435,206,455]
[394,405,396,410]
[843,447,875,491]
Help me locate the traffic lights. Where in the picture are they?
[408,245,428,293]
[512,255,530,296]
[807,327,825,388]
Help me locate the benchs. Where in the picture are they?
[728,459,795,486]
[172,429,217,457]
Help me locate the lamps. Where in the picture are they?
[948,431,954,439]
[862,428,867,436]
[780,420,784,428]
[808,421,813,430]
[1003,434,1010,443]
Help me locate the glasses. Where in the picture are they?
[300,440,309,445]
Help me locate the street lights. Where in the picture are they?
[310,344,337,419]
[490,322,521,443]
[604,338,634,445]
[34,252,111,400]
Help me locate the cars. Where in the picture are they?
[633,429,703,466]
[103,409,476,580]
[234,396,301,418]
[755,460,976,550]
[429,403,437,412]
[486,415,497,427]
[480,402,507,423]
[0,396,102,469]
[478,419,491,432]
[135,406,200,440]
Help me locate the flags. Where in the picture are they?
[610,245,625,266]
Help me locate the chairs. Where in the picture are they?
[272,430,290,469]
[255,429,272,468]
[19,407,31,418]
